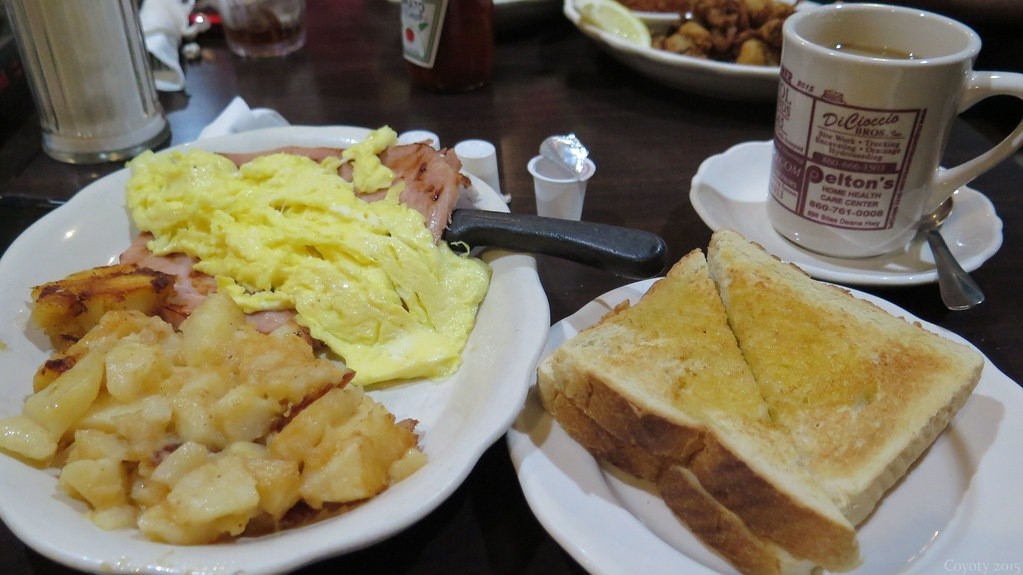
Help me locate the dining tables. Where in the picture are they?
[0,0,1023,575]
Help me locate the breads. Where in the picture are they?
[538,229,985,575]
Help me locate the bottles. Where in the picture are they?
[404,1,498,93]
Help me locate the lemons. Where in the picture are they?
[582,0,651,48]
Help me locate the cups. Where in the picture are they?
[0,0,172,164]
[769,4,1023,257]
[528,156,595,223]
[217,0,310,57]
[400,131,502,199]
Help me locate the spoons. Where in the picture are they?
[919,194,986,312]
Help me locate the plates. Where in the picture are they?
[505,277,1022,575]
[562,0,827,96]
[688,138,1004,282]
[1,127,552,575]
